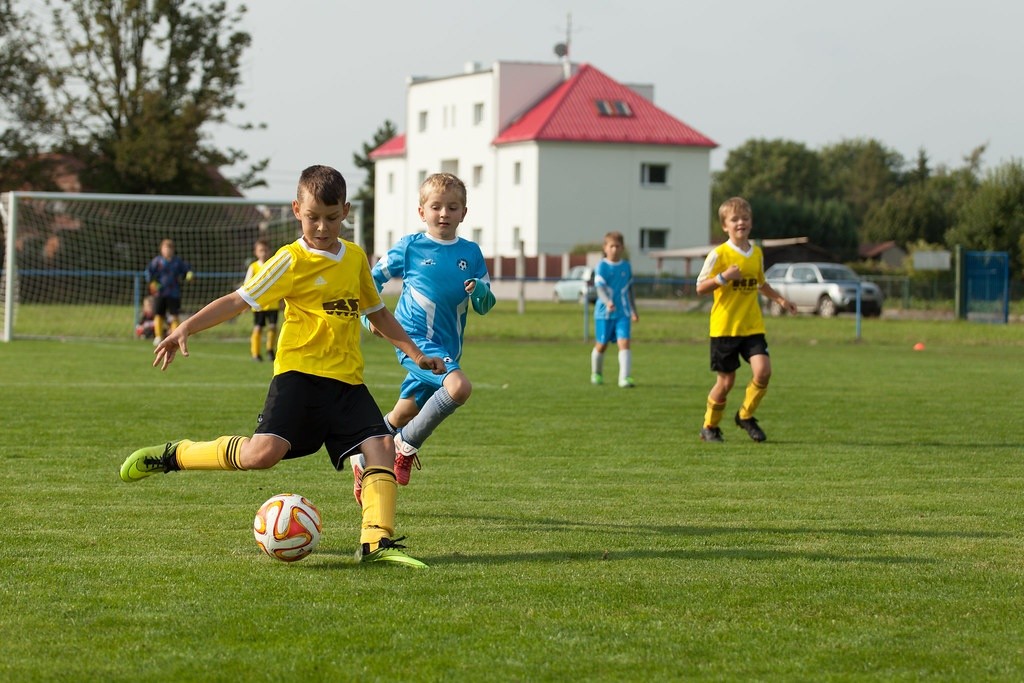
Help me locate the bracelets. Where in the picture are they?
[717,273,728,284]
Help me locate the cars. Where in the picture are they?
[759,260,884,319]
[553,266,600,307]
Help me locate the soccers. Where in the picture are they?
[254,494,325,561]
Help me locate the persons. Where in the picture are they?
[590,231,638,388]
[243,240,280,362]
[696,197,798,444]
[348,173,497,507]
[135,238,194,346]
[118,164,448,569]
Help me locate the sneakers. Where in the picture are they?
[266,350,276,361]
[153,337,161,346]
[253,355,262,363]
[119,439,182,483]
[357,536,430,570]
[591,374,602,385]
[348,453,366,507]
[699,426,725,442]
[393,431,421,486]
[734,410,767,442]
[617,377,635,388]
